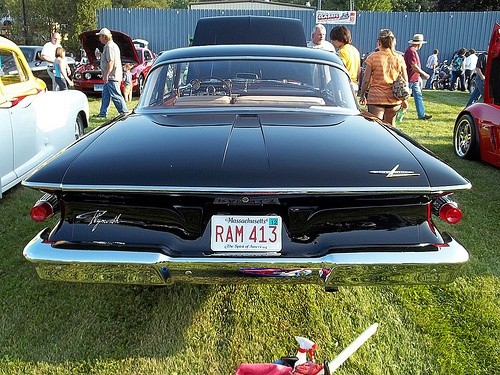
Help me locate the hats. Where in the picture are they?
[378,29,394,38]
[95,28,112,37]
[408,33,427,44]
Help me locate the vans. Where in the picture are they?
[451,21,500,169]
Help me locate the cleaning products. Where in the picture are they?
[291,336,316,373]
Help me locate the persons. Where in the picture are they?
[359,27,410,125]
[122,63,134,102]
[92,27,129,118]
[307,23,335,89]
[425,49,440,89]
[447,47,488,107]
[326,26,361,98]
[403,33,432,120]
[54,46,74,91]
[40,32,62,91]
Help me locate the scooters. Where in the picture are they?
[430,58,452,91]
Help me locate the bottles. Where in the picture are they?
[95,48,100,60]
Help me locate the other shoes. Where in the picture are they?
[421,114,431,120]
[92,114,105,118]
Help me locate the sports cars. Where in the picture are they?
[20,14,473,287]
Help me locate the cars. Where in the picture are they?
[0,33,47,110]
[3,45,81,91]
[0,88,91,199]
[69,30,158,97]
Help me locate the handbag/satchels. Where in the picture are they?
[392,73,411,100]
